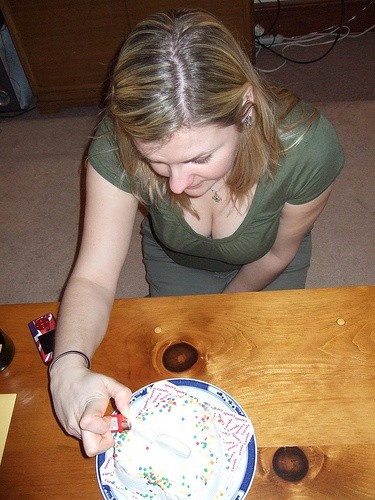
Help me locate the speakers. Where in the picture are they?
[0,20,32,118]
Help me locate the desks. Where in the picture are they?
[0,283,375,500]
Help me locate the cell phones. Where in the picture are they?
[28,312,57,367]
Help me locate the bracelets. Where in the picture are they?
[48,350,90,372]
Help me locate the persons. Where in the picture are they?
[50,8,346,457]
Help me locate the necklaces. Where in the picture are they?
[210,184,225,202]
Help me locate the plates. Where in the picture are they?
[96,378,257,500]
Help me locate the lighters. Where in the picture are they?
[109,414,131,434]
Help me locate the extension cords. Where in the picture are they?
[254,34,284,46]
